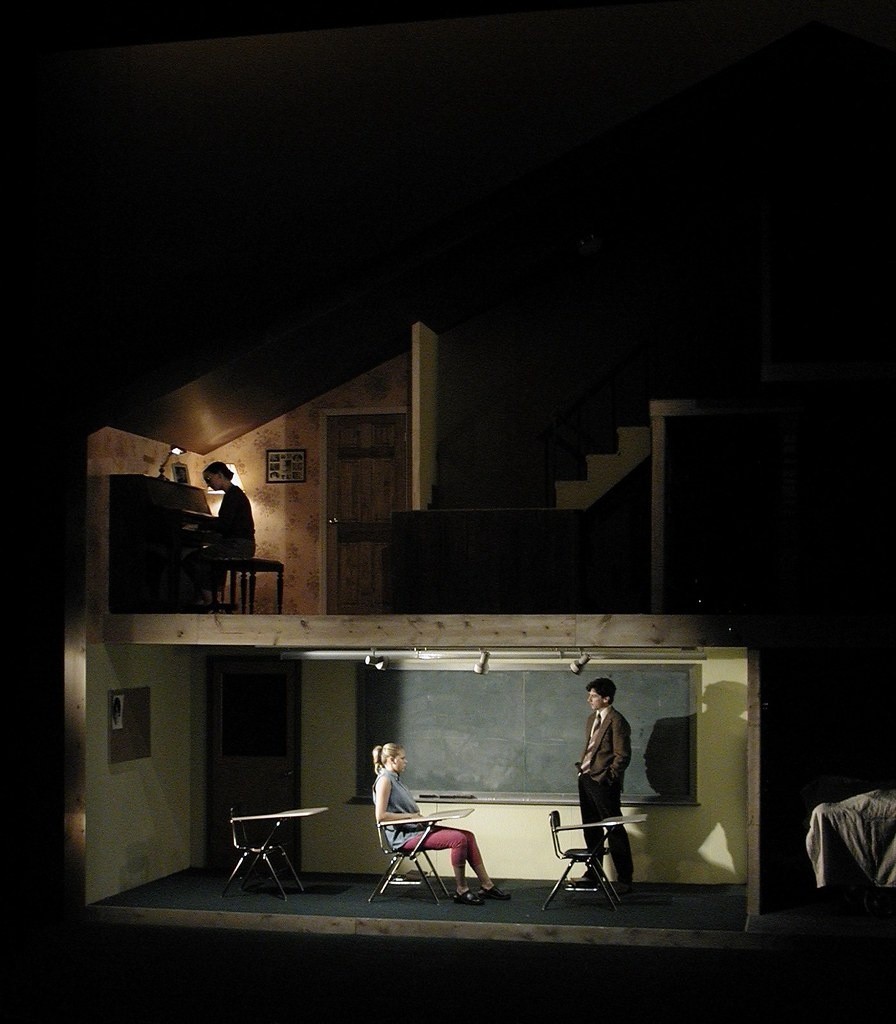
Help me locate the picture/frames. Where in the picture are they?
[172,461,191,485]
[266,449,305,483]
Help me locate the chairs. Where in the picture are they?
[220,807,329,902]
[541,811,648,912]
[368,808,476,904]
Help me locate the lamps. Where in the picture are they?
[570,654,591,676]
[473,651,491,675]
[365,655,389,671]
[208,464,245,495]
[157,445,187,482]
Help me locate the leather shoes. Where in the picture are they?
[453,889,485,906]
[478,886,511,900]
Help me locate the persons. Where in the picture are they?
[372,743,511,905]
[185,462,255,613]
[564,678,633,896]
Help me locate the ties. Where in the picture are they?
[581,714,601,774]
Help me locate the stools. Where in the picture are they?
[211,557,284,614]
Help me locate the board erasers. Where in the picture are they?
[419,792,475,800]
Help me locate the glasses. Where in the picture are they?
[205,475,212,483]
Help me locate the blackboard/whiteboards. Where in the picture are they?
[348,661,705,808]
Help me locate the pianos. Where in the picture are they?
[110,474,211,615]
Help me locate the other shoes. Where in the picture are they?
[617,884,632,896]
